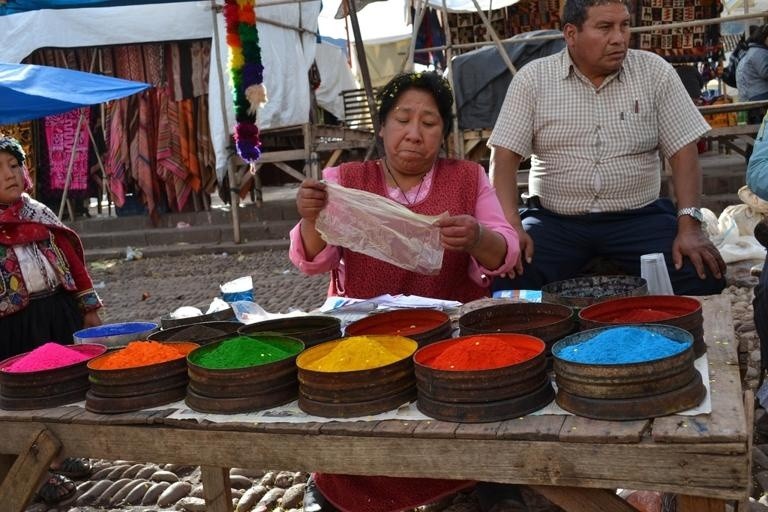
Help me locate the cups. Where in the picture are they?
[639,252,676,298]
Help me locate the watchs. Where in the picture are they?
[676,207,703,222]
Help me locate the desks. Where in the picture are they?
[2,295,755,511]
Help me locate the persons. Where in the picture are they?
[746,110,768,381]
[0,132,104,507]
[723,22,768,102]
[485,1,729,296]
[286,71,520,511]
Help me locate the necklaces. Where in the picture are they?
[382,156,427,205]
